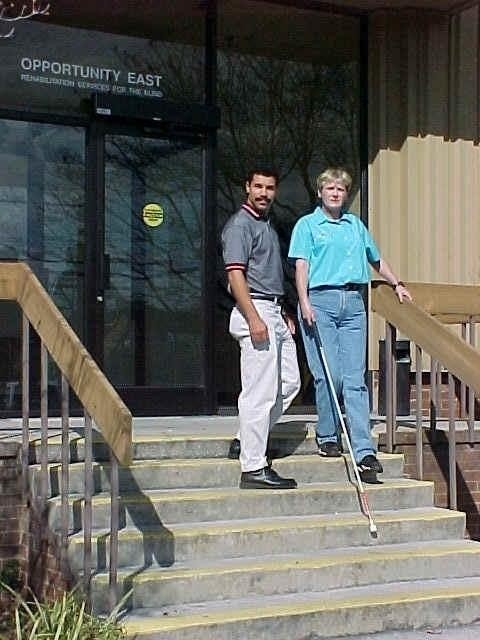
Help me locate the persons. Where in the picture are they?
[287,164,415,475]
[220,163,304,491]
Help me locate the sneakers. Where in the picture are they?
[357,456,384,474]
[318,441,342,458]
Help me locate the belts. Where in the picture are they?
[307,283,362,292]
[251,293,284,304]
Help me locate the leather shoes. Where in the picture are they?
[229,439,241,459]
[239,468,298,490]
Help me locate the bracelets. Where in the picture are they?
[391,281,407,291]
